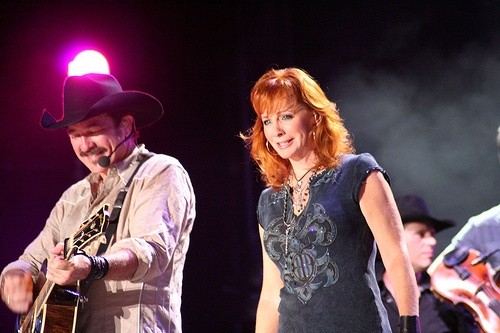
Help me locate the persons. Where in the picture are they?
[240,68,421,333]
[427,204,500,276]
[377,195,480,333]
[0,75,196,333]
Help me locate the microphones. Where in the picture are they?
[98,135,130,167]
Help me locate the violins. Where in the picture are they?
[430,246,500,332]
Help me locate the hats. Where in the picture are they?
[40,72,163,130]
[397,194,456,230]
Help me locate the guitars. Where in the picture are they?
[14,205,110,333]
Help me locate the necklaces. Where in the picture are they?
[284,166,322,255]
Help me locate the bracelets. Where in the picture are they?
[79,254,109,310]
